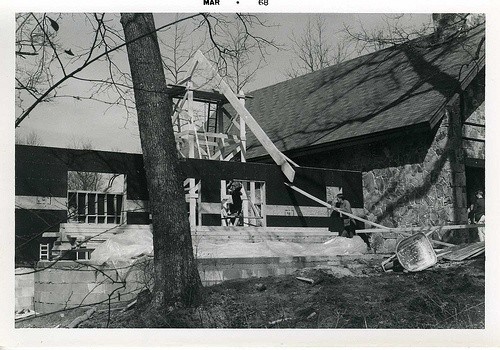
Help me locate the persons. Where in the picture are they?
[468,190,485,241]
[68,203,77,222]
[331,194,355,238]
[232,182,243,227]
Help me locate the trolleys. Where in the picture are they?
[381,226,452,274]
